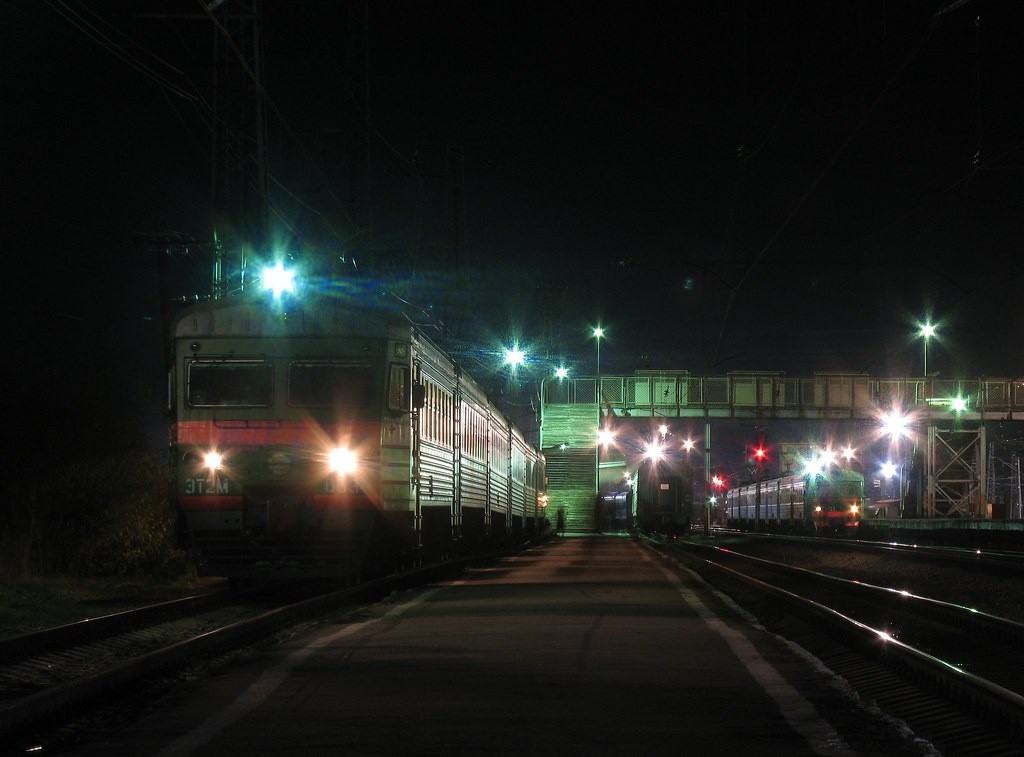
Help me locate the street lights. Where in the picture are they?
[593,322,605,376]
[922,325,937,377]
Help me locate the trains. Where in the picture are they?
[723,466,865,540]
[163,278,547,583]
[601,454,694,537]
